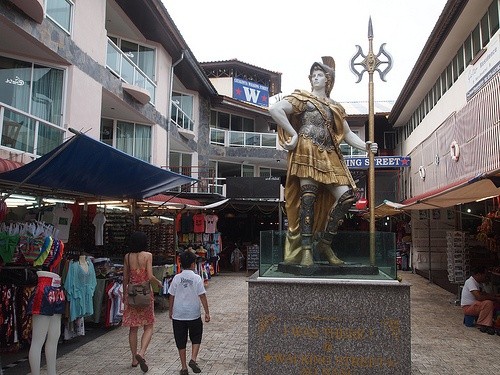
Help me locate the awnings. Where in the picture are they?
[359,172,500,227]
[0,127,231,218]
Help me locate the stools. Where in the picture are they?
[463,316,478,327]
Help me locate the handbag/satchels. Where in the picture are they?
[130,281,150,308]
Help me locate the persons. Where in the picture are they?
[267,56,378,265]
[168,251,211,375]
[29,270,68,375]
[461,266,496,335]
[123,232,164,373]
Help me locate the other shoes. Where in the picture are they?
[189,360,201,373]
[180,369,189,375]
[480,327,495,335]
[132,361,138,367]
[136,354,148,372]
